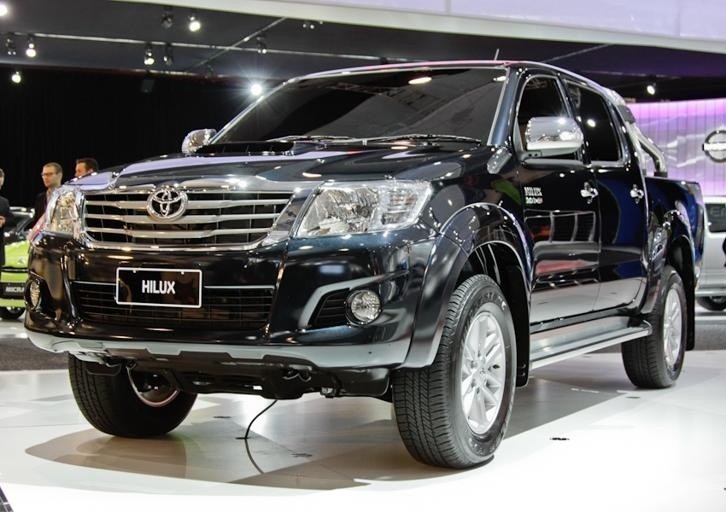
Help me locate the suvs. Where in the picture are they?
[24,59,708,468]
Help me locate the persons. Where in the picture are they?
[33,161,63,225]
[73,157,99,178]
[0,168,18,280]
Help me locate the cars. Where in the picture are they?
[694,195,726,313]
[0,207,37,320]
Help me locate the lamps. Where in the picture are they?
[143,4,200,69]
[5,32,37,58]
[606,77,659,96]
[185,18,318,79]
